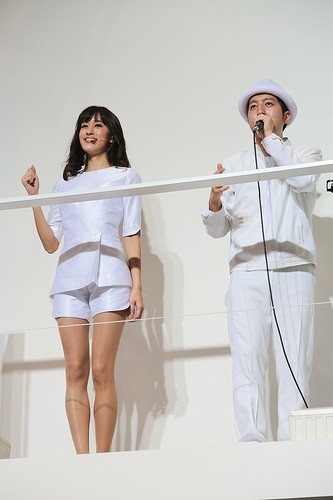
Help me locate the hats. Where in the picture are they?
[237,79,298,128]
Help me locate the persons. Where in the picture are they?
[202,79,324,443]
[21,106,144,453]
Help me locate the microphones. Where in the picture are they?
[253,120,264,131]
[90,139,109,144]
